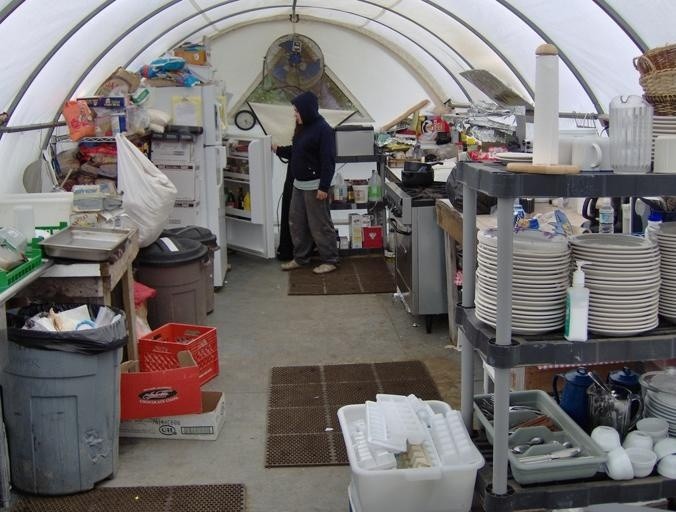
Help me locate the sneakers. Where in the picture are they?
[313,262,338,274]
[281,259,302,270]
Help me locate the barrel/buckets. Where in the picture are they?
[141,238,208,328]
[334,173,347,202]
[168,225,221,315]
[353,184,367,204]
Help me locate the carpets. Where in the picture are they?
[263,361,448,468]
[286,256,397,295]
[2,482,248,511]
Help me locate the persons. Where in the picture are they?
[269,92,341,275]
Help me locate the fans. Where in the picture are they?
[269,34,325,93]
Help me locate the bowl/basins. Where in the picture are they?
[656,454,676,480]
[636,416,670,439]
[602,445,635,480]
[652,437,676,462]
[590,426,621,452]
[622,430,653,451]
[623,446,658,478]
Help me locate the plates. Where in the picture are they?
[638,366,676,437]
[650,115,676,169]
[472,218,676,337]
[494,156,533,165]
[493,152,533,165]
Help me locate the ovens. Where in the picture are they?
[388,218,447,315]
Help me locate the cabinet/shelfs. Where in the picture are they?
[456,163,676,511]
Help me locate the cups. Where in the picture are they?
[654,133,676,175]
[605,367,642,394]
[571,135,602,172]
[552,366,601,431]
[585,379,644,442]
[608,90,654,176]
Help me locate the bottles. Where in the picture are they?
[644,213,664,241]
[237,186,245,210]
[334,229,341,249]
[598,196,614,233]
[346,181,355,203]
[225,190,236,209]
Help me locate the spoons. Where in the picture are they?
[510,435,584,463]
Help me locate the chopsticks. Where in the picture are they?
[508,414,562,432]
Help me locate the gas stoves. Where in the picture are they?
[382,180,450,218]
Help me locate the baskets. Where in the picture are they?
[0,248,43,293]
[633,44,676,115]
[138,322,219,388]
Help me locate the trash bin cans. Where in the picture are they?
[134,225,222,342]
[0,303,129,495]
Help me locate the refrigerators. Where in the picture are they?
[140,83,278,287]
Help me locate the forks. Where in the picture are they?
[480,392,543,420]
